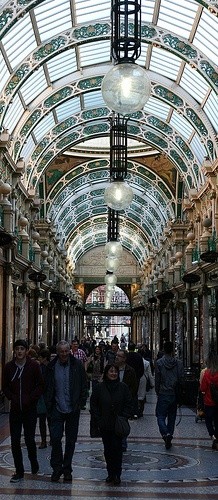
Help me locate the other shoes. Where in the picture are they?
[64,471,72,480]
[133,415,138,419]
[81,405,86,410]
[10,472,24,483]
[212,439,218,450]
[114,475,121,484]
[39,441,47,449]
[106,474,113,481]
[164,433,172,449]
[51,467,63,481]
[30,458,39,474]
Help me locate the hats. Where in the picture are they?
[14,339,27,350]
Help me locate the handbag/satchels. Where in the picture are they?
[87,361,94,373]
[174,381,185,405]
[146,379,150,392]
[116,415,130,435]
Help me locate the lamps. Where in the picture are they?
[101,0,151,113]
[104,109,133,212]
[103,207,121,309]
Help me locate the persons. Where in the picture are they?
[89,363,131,484]
[43,340,90,484]
[154,341,184,449]
[69,334,154,420]
[201,369,218,451]
[156,346,164,360]
[2,339,44,482]
[199,367,209,410]
[26,342,57,449]
